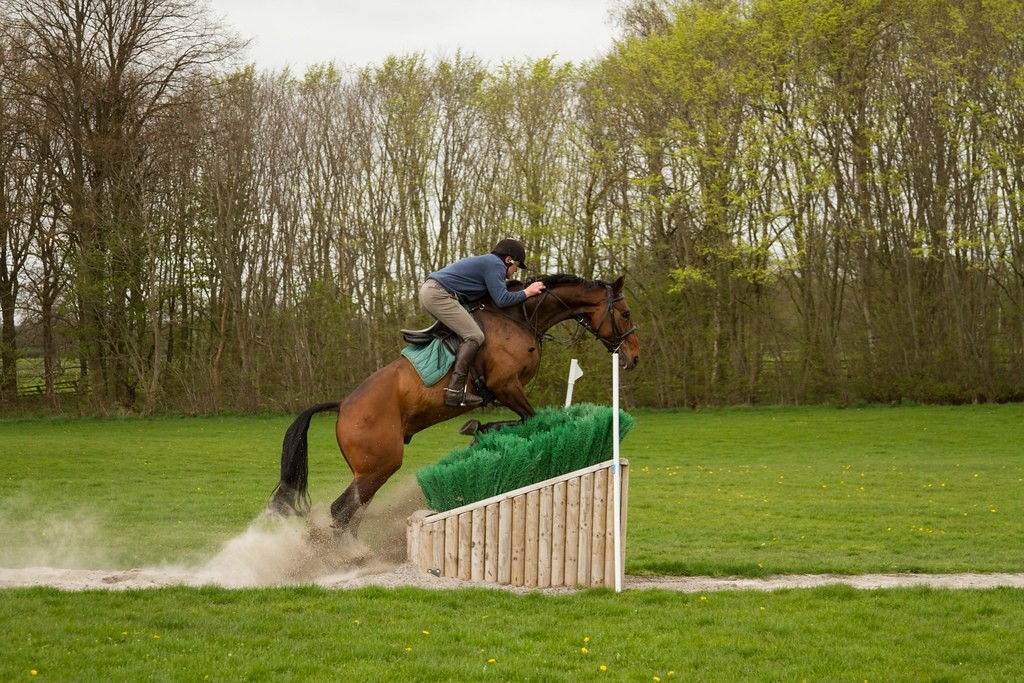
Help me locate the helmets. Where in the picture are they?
[493,239,526,269]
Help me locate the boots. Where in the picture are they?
[445,337,483,406]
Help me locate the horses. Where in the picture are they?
[264,272,641,540]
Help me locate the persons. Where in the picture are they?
[419,239,546,407]
